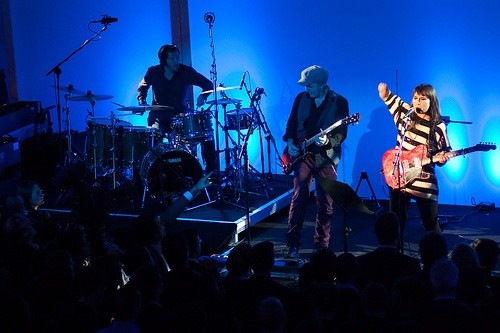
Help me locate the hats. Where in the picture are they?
[297,65,328,86]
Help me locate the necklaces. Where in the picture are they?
[414,115,426,125]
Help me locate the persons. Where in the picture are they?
[378,81,451,250]
[137,45,213,145]
[0,158,500,333]
[282,64,349,255]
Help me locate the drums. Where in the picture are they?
[85,118,132,169]
[171,111,214,142]
[139,142,205,208]
[225,108,258,130]
[122,125,162,170]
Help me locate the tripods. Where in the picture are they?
[45,22,283,214]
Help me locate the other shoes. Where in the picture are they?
[311,242,323,255]
[280,243,299,258]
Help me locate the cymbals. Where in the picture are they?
[206,98,243,104]
[199,86,243,94]
[50,85,85,95]
[66,95,114,101]
[116,105,175,111]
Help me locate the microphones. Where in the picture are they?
[403,107,415,119]
[250,92,257,107]
[99,17,118,23]
[240,71,246,89]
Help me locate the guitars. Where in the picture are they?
[382,141,497,190]
[281,112,360,175]
